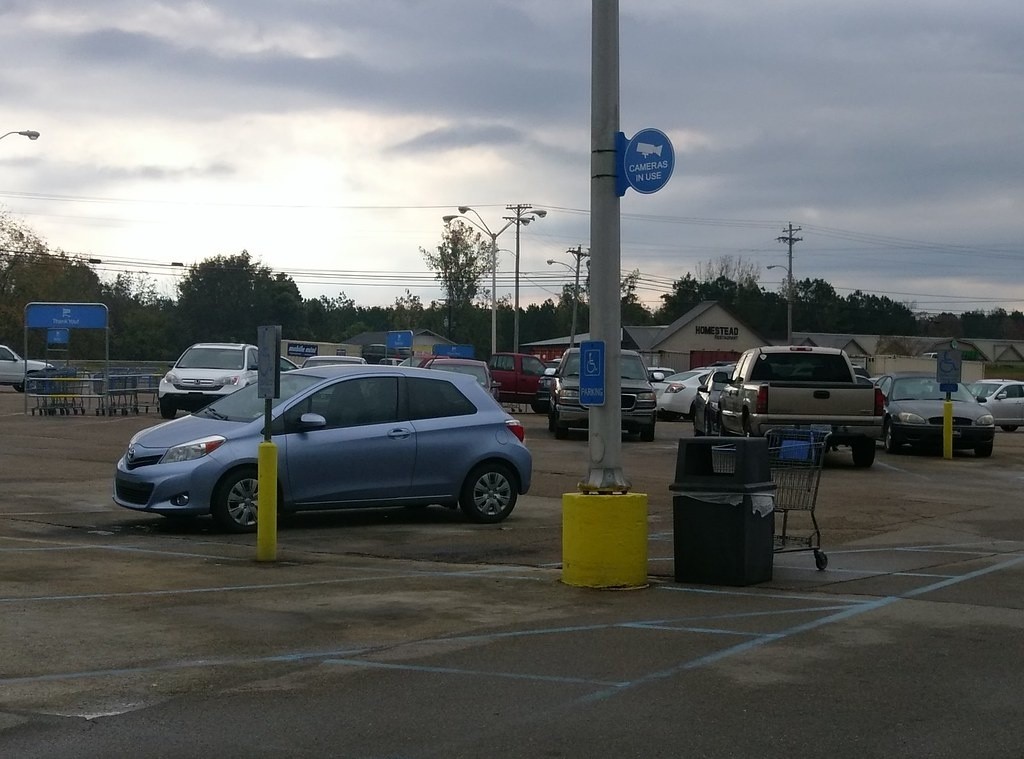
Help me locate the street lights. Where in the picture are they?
[767,265,793,344]
[547,259,580,348]
[442,205,547,367]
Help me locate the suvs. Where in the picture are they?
[544,346,666,442]
[158,343,259,418]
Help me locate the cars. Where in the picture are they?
[302,355,368,371]
[111,361,532,533]
[533,357,563,407]
[966,381,1024,431]
[850,361,880,386]
[0,345,56,392]
[397,355,450,367]
[380,358,404,367]
[279,355,302,374]
[423,359,502,404]
[874,371,996,456]
[646,366,680,422]
[650,369,712,422]
[692,367,736,436]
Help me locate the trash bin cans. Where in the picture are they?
[668,435,779,586]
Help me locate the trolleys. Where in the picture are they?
[27,364,164,416]
[761,427,832,571]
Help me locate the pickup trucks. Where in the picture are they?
[487,352,549,414]
[719,345,887,469]
